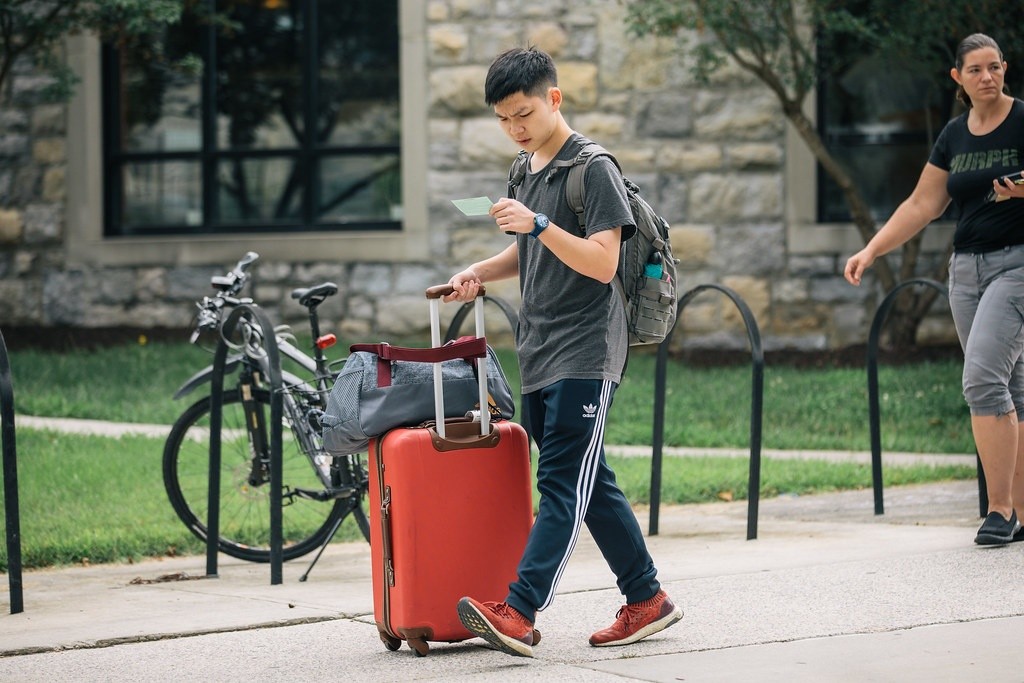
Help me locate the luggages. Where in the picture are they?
[367,281,541,658]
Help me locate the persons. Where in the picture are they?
[843,31,1024,543]
[440,47,688,658]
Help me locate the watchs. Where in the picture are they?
[528,212,550,238]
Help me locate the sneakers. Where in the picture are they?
[456,596,535,657]
[588,587,684,647]
[974,507,1024,544]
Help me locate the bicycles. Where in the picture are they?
[161,251,374,582]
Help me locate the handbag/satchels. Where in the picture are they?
[317,336,516,455]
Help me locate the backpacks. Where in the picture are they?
[545,143,681,346]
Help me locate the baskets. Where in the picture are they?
[274,358,348,456]
